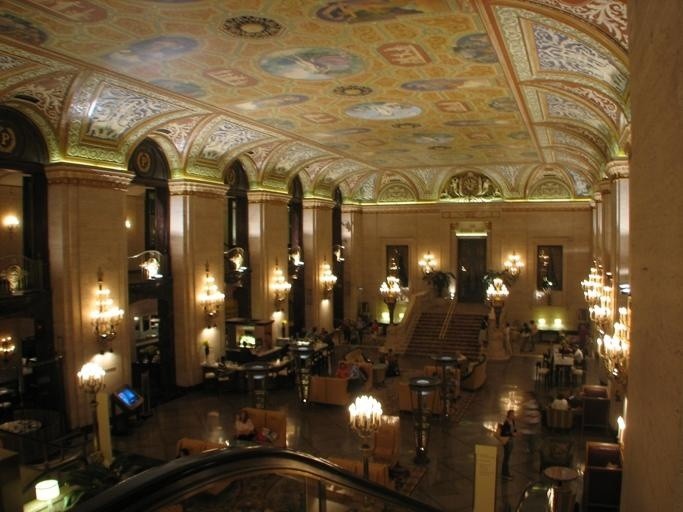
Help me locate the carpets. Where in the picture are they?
[370,368,478,426]
[325,457,427,496]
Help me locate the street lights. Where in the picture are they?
[2,213,26,396]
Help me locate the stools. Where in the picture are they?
[537,368,550,394]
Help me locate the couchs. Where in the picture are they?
[308,347,488,416]
[174,407,287,502]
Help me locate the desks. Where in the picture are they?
[552,343,575,387]
[544,466,577,499]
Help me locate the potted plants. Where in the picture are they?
[423,270,456,297]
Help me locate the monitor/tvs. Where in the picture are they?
[109,384,144,411]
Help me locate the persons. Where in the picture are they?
[431,350,473,404]
[573,343,584,365]
[551,389,578,410]
[478,315,538,355]
[217,316,400,389]
[235,410,257,441]
[500,390,541,480]
